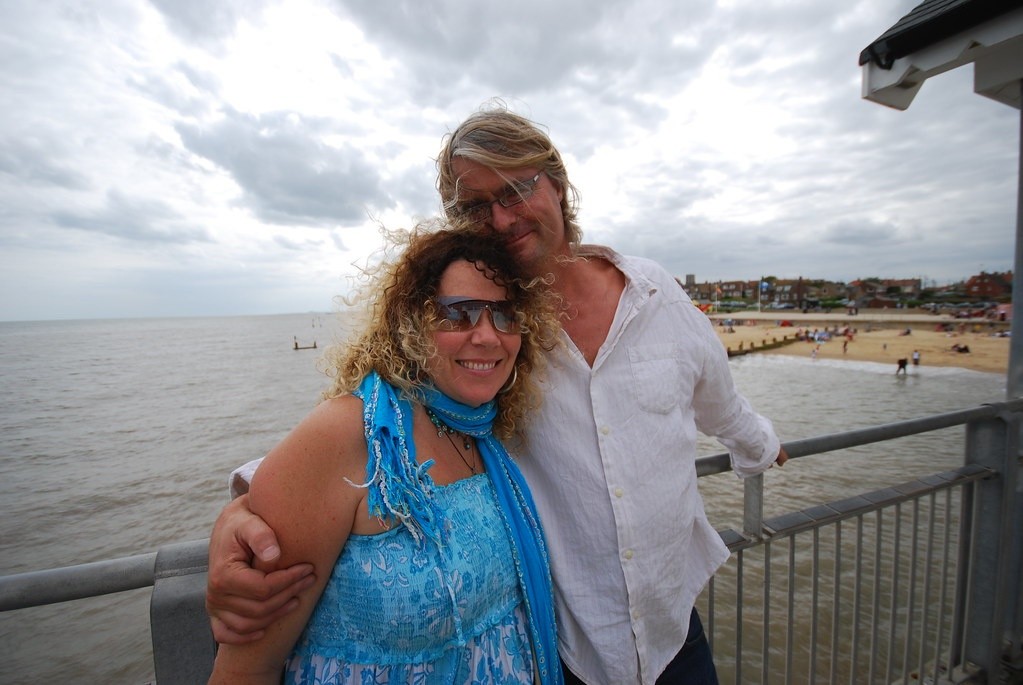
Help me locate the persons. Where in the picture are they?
[797,321,856,358]
[207,110,788,685]
[206,224,567,685]
[953,344,969,353]
[912,350,920,365]
[896,357,908,374]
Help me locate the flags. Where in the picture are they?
[759,281,771,289]
[717,287,722,293]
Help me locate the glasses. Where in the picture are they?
[464,168,545,223]
[433,295,515,333]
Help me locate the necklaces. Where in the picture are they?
[426,408,480,476]
